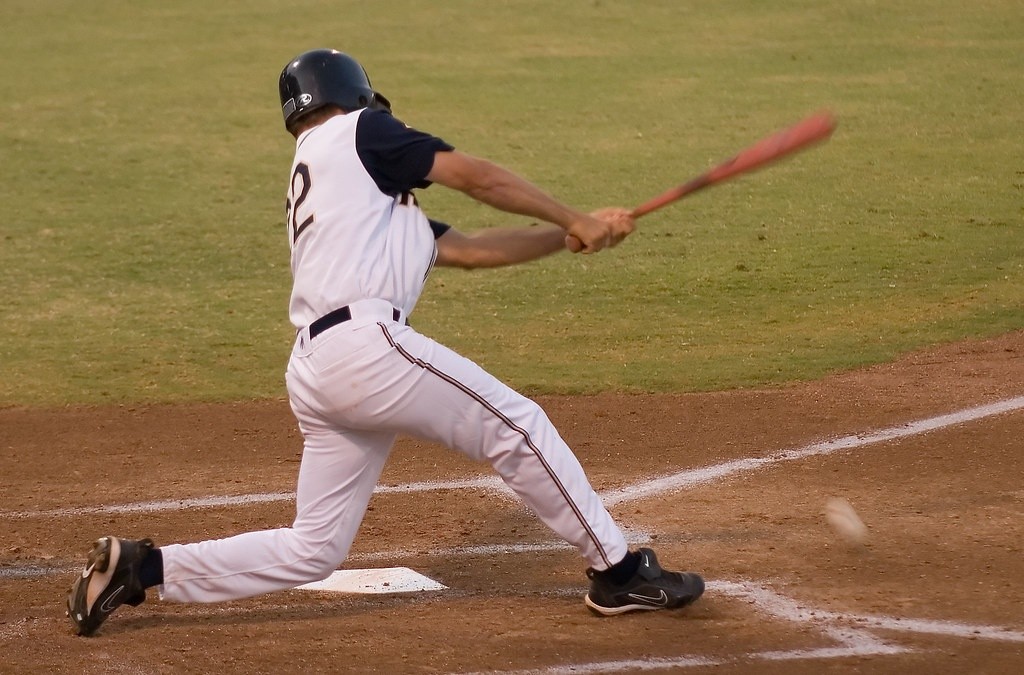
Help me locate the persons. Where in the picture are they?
[64,49,706,636]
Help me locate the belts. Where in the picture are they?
[308,300,410,339]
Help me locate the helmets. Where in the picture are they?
[279,49,391,132]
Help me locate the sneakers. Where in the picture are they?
[583,548,705,618]
[66,536,155,637]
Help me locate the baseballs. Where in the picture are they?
[828,496,867,545]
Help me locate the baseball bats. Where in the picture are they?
[565,110,839,254]
